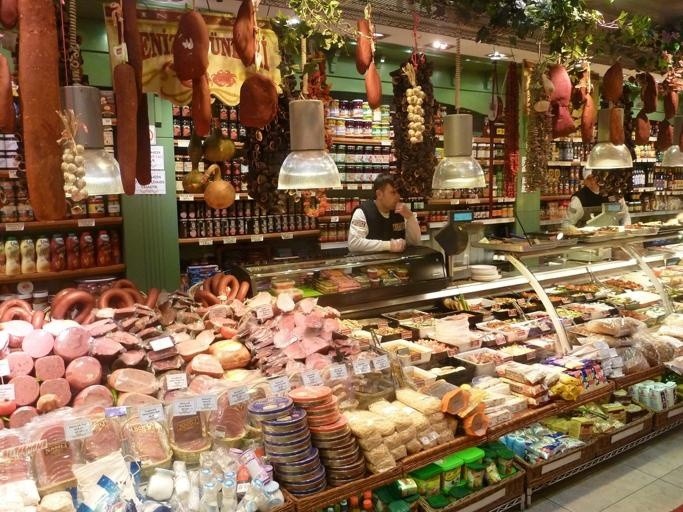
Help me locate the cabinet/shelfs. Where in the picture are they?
[1,131,682,511]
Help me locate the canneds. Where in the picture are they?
[71,194,121,220]
[173,99,514,243]
[0,133,34,223]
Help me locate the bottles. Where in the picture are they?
[624,193,682,212]
[196,466,262,512]
[546,142,596,161]
[0,229,126,274]
[629,164,682,192]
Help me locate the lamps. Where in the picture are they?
[428,23,487,192]
[276,35,344,192]
[59,0,127,199]
[658,98,682,171]
[582,102,635,172]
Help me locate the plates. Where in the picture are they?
[470,264,501,281]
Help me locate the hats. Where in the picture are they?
[581,165,596,180]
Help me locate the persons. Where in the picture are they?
[347,173,423,253]
[559,170,631,235]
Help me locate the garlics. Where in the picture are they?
[60,143,88,202]
